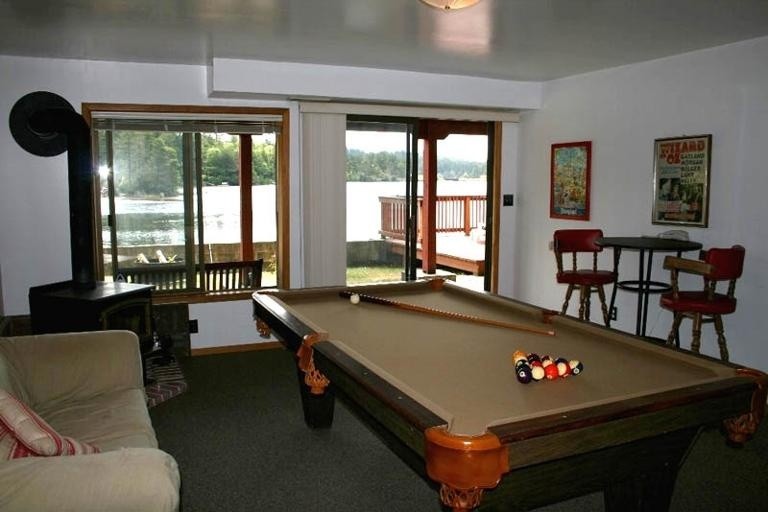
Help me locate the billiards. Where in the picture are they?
[350,294,360,304]
[513,350,583,383]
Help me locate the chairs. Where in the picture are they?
[660,243,745,360]
[553,229,616,328]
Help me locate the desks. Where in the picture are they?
[250,277,767,511]
[596,236,703,336]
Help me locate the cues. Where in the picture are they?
[339,290,555,336]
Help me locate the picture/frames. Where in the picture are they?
[549,141,592,221]
[651,133,712,227]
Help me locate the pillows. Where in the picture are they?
[0,389,101,455]
[0,423,37,459]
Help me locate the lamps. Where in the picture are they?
[420,0,479,11]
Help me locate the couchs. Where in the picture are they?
[1,331,181,512]
